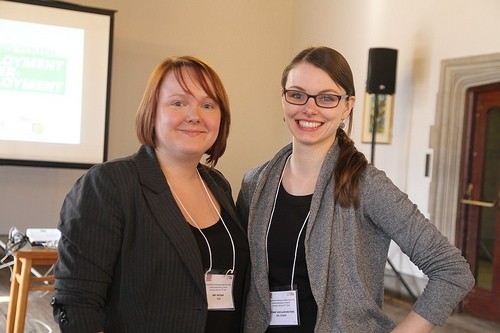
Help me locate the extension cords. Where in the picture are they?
[9,239,32,252]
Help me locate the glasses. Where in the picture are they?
[284,89,348,108]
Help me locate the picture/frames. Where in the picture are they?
[362,94,395,144]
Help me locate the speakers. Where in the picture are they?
[365,48,398,94]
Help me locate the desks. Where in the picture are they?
[0,235,58,333]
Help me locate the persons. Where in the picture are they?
[235,46,476,333]
[50,55,250,333]
